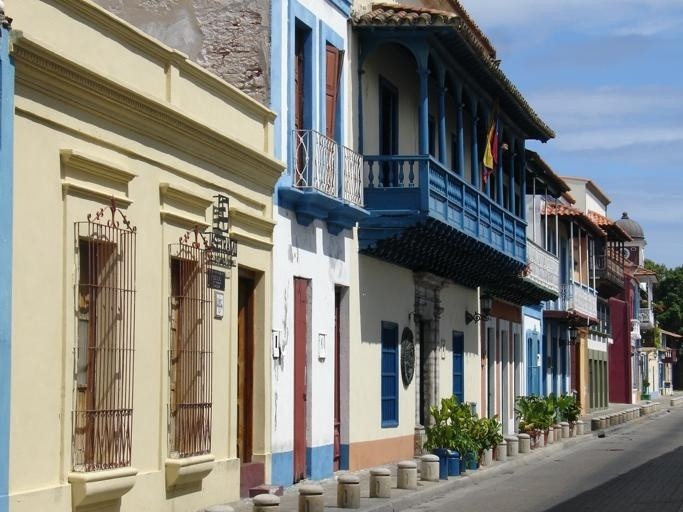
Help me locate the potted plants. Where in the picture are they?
[513,388,583,450]
[420,393,506,481]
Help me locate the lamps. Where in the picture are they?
[559,321,580,348]
[632,344,643,357]
[464,292,493,326]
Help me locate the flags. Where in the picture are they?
[481,97,500,185]
